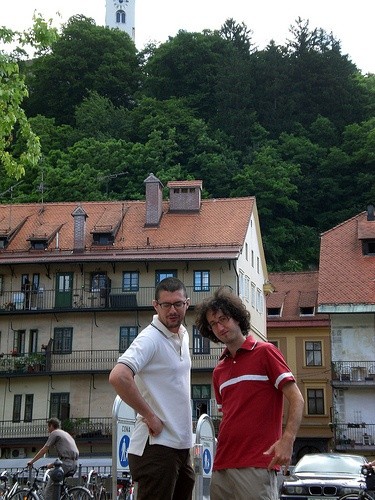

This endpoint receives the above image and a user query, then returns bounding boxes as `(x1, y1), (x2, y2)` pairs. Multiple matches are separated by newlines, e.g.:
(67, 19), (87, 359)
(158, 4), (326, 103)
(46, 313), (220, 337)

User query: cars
(277, 452), (369, 500)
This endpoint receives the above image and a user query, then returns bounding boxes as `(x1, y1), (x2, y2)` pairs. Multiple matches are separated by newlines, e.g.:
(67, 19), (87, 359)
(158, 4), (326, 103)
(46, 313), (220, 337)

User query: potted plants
(61, 416), (89, 439)
(10, 349), (45, 374)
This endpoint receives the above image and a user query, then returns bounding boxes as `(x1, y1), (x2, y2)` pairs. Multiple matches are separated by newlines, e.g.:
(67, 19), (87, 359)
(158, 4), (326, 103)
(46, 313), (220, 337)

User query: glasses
(156, 300), (186, 310)
(208, 316), (231, 330)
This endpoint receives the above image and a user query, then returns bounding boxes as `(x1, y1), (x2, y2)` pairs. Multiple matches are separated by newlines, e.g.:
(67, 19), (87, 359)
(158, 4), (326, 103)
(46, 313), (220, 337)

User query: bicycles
(337, 464), (375, 500)
(0, 463), (135, 500)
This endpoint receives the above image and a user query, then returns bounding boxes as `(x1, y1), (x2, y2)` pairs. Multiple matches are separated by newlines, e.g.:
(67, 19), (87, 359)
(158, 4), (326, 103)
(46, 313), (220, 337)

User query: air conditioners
(350, 367), (367, 383)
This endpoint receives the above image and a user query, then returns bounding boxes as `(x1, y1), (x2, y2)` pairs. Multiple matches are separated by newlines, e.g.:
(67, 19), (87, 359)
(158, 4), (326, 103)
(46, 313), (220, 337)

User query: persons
(109, 276), (194, 500)
(196, 290), (305, 500)
(361, 462), (375, 491)
(27, 417), (79, 500)
(21, 284), (29, 299)
(104, 276), (112, 293)
